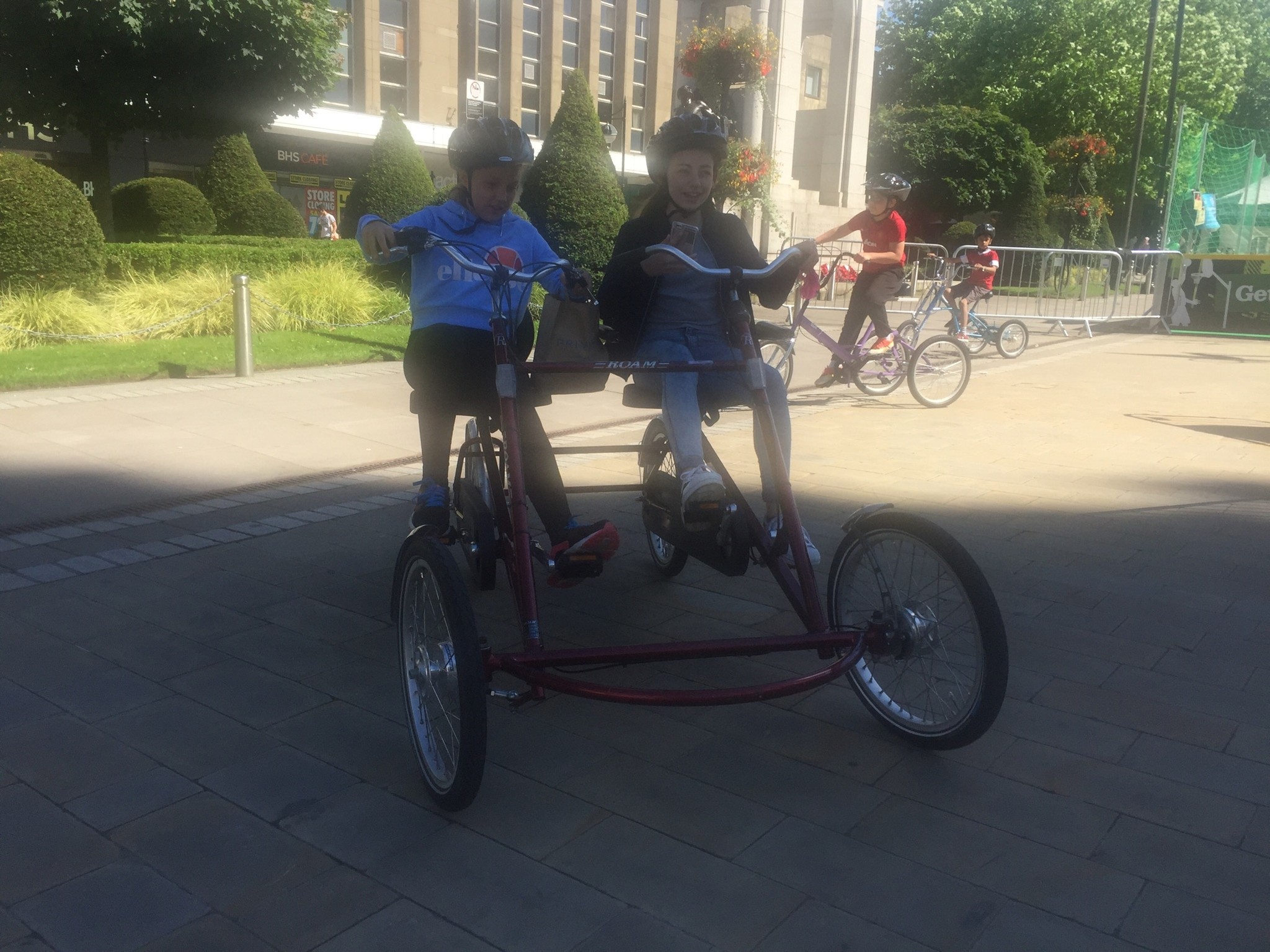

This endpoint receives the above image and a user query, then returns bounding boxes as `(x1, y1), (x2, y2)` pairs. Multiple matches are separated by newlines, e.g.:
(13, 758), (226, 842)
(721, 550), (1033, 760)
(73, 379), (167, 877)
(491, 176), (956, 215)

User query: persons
(315, 203), (338, 240)
(617, 113), (821, 564)
(670, 85), (719, 121)
(358, 115), (620, 588)
(808, 173), (912, 386)
(927, 223), (1000, 341)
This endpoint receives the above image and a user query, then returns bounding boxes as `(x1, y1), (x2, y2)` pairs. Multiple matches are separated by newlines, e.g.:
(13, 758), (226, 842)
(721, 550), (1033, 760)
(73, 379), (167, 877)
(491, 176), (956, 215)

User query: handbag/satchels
(534, 272), (610, 393)
(332, 232), (339, 240)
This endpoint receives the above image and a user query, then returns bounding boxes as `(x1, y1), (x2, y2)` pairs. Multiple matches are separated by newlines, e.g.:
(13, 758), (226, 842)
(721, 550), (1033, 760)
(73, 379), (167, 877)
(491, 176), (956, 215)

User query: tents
(1199, 172), (1270, 255)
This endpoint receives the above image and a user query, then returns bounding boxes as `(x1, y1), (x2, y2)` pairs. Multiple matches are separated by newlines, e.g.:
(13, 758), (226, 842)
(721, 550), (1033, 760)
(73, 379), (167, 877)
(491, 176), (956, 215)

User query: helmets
(975, 224), (996, 239)
(449, 116), (535, 169)
(645, 113), (730, 185)
(861, 172), (912, 201)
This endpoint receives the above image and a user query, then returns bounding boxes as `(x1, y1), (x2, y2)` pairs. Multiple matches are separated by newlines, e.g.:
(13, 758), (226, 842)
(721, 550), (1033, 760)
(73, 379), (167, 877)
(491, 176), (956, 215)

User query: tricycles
(890, 254), (1029, 362)
(754, 251), (973, 410)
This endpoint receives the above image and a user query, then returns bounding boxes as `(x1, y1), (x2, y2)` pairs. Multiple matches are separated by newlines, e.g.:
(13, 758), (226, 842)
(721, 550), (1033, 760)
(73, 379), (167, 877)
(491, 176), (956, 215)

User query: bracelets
(982, 266), (986, 272)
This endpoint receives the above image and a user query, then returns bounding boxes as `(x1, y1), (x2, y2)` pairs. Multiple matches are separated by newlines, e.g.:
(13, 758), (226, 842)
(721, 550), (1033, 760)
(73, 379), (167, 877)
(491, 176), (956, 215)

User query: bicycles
(388, 226), (1013, 814)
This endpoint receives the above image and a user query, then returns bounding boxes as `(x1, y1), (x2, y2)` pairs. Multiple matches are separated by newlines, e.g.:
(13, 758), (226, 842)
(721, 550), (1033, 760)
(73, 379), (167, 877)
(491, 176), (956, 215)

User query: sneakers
(866, 332), (895, 355)
(764, 512), (821, 566)
(680, 463), (726, 532)
(409, 477), (451, 538)
(815, 366), (838, 385)
(954, 333), (969, 341)
(546, 517), (620, 588)
(945, 314), (961, 327)
